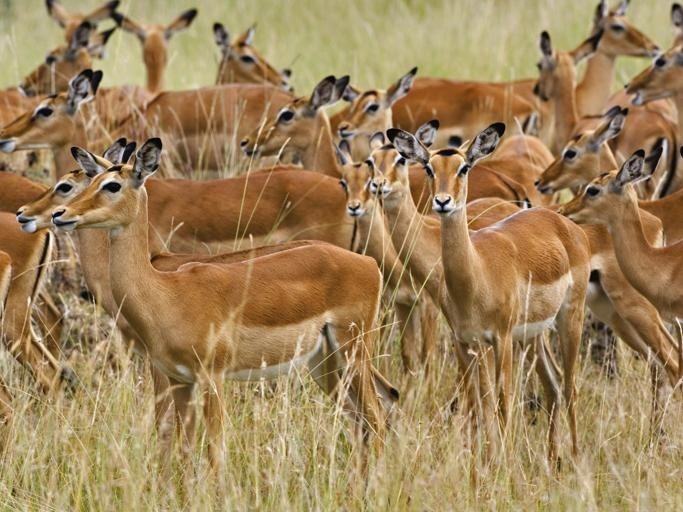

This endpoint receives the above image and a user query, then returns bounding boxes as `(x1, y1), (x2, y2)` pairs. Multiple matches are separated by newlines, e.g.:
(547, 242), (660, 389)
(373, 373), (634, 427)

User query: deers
(1, 0), (683, 478)
